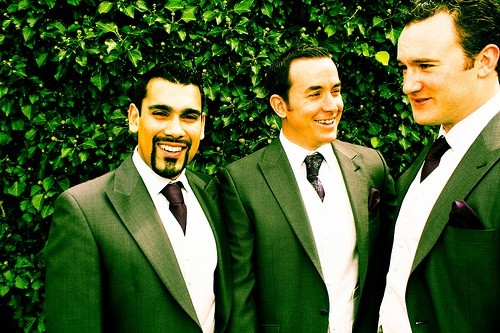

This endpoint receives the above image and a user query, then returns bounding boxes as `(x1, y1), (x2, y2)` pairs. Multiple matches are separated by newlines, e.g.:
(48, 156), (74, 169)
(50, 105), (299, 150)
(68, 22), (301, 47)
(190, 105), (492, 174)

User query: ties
(160, 183), (186, 236)
(304, 154), (325, 203)
(420, 134), (452, 181)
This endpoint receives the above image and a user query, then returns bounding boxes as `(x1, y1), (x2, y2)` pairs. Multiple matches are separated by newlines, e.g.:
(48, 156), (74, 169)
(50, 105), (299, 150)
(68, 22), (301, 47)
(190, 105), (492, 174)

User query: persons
(45, 61), (232, 333)
(353, 0), (500, 333)
(215, 45), (397, 333)
